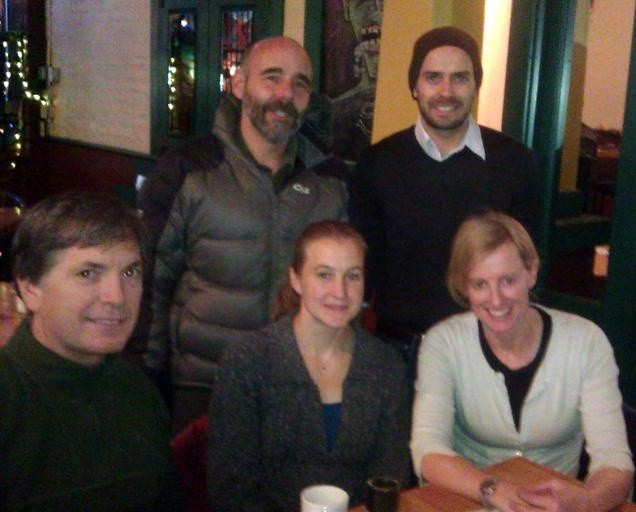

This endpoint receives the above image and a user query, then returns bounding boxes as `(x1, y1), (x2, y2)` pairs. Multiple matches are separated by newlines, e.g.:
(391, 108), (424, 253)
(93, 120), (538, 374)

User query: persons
(127, 33), (358, 428)
(405, 207), (636, 512)
(1, 190), (189, 512)
(205, 218), (413, 512)
(349, 23), (542, 366)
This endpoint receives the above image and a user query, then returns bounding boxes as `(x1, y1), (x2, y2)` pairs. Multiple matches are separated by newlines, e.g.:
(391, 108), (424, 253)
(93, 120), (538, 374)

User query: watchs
(479, 477), (504, 511)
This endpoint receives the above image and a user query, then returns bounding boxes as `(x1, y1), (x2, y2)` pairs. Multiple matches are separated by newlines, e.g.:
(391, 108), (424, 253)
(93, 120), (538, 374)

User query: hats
(409, 27), (482, 92)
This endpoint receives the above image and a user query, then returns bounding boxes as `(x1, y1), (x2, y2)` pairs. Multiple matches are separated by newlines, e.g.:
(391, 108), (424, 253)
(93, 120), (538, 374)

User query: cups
(298, 477), (400, 512)
(592, 244), (609, 277)
